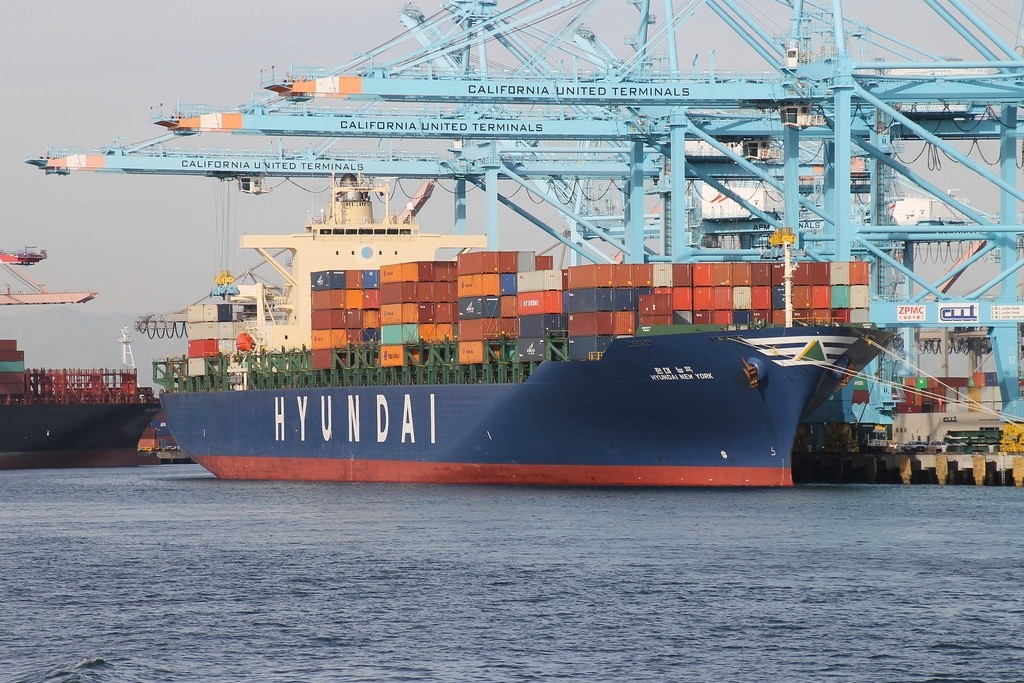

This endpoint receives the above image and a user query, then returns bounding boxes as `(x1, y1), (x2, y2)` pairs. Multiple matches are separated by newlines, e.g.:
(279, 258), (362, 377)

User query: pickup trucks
(901, 439), (946, 453)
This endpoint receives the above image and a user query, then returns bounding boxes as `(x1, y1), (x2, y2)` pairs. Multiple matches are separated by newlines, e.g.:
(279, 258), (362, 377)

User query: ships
(151, 322), (897, 490)
